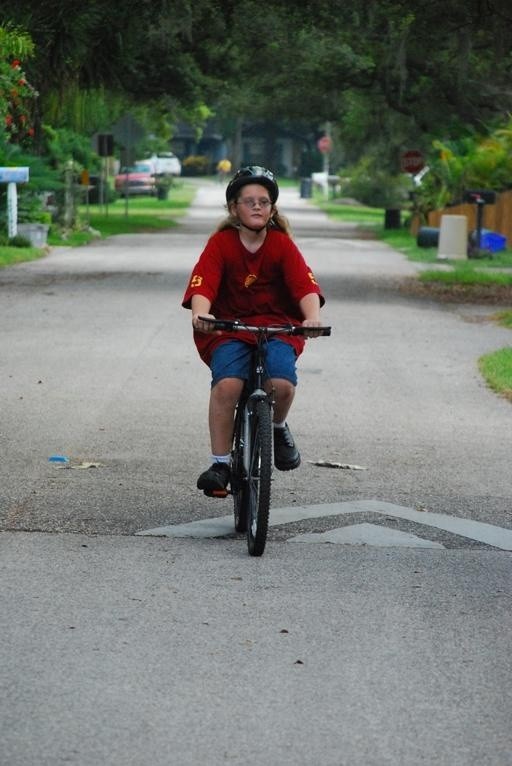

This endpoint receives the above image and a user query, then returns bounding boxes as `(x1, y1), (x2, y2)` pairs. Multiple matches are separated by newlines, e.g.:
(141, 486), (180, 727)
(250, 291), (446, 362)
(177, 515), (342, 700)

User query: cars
(113, 163), (155, 198)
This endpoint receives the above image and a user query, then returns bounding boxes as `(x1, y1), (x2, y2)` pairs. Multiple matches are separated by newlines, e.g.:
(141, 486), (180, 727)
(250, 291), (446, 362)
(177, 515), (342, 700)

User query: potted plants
(17, 196), (51, 248)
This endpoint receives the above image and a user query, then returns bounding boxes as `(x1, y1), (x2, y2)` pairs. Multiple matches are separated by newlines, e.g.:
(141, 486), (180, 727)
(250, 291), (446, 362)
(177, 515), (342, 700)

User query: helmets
(226, 165), (278, 205)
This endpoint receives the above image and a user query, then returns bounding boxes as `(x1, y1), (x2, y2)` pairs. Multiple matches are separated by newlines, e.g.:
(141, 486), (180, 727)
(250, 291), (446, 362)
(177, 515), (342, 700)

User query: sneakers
(274, 421), (301, 471)
(196, 462), (231, 490)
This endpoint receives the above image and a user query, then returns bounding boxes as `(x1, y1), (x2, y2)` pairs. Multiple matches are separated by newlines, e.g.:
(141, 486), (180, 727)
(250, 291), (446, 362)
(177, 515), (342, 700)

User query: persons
(216, 159), (233, 182)
(181, 164), (325, 488)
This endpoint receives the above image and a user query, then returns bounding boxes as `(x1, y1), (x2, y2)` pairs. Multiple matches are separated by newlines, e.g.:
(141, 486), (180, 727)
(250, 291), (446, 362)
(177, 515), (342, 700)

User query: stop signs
(400, 148), (424, 175)
(318, 137), (334, 153)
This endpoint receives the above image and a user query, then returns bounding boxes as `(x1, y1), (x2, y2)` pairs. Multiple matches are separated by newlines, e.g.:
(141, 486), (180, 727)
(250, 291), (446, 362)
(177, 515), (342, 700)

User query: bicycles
(191, 308), (334, 562)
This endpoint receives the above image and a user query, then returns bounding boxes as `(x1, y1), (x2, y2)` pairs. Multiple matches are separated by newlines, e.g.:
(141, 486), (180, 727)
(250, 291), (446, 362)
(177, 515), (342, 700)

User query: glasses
(236, 198), (273, 209)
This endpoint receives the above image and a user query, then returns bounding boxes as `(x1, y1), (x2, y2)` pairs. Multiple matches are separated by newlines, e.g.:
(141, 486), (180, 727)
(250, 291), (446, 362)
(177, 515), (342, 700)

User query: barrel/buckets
(469, 229), (508, 254)
(415, 225), (440, 248)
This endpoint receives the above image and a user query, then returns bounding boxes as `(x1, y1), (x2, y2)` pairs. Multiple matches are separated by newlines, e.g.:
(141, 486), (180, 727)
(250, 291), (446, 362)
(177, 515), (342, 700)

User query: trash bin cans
(158, 184), (168, 199)
(300, 177), (313, 198)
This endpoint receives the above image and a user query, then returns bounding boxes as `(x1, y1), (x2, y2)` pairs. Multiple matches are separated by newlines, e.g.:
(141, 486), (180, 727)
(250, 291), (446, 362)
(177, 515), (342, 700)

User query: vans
(132, 149), (183, 176)
